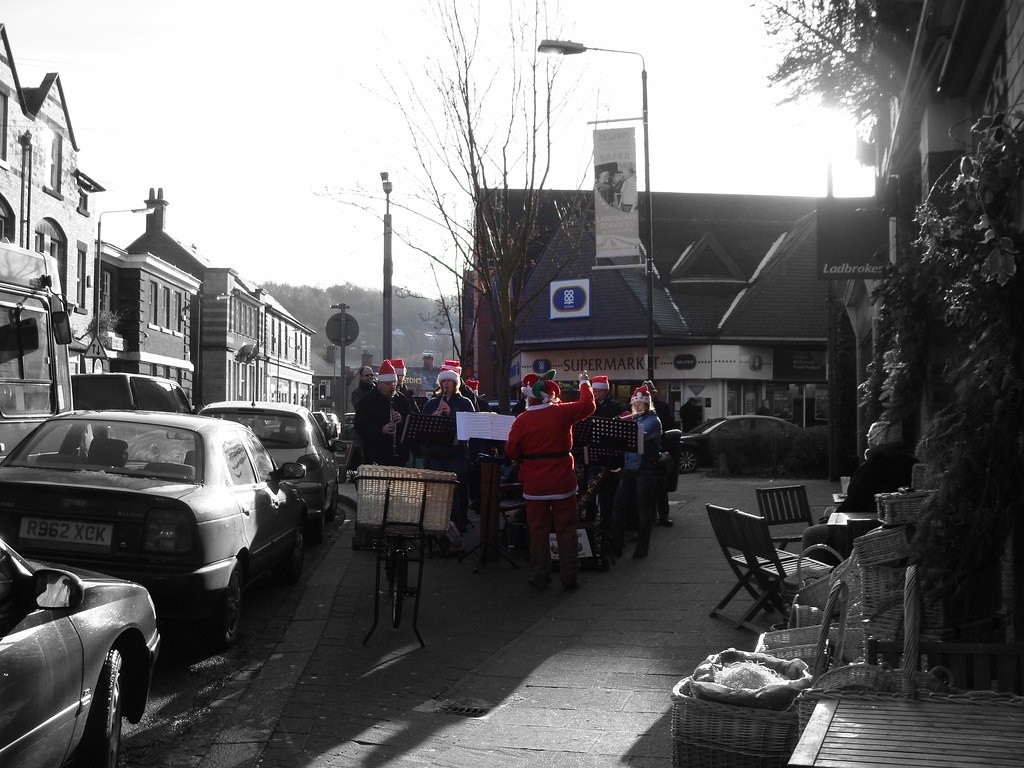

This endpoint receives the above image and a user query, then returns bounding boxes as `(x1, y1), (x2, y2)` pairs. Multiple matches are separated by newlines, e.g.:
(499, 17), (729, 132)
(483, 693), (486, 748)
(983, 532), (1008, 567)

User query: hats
(379, 359), (397, 381)
(630, 385), (651, 403)
(437, 361), (460, 387)
(465, 379), (479, 394)
(526, 380), (561, 410)
(592, 376), (609, 390)
(390, 359), (407, 375)
(643, 381), (657, 392)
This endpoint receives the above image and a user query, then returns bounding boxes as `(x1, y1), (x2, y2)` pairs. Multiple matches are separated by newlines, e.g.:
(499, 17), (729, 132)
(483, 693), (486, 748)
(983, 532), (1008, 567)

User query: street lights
(277, 329), (302, 402)
(537, 39), (655, 393)
(197, 292), (229, 402)
(381, 172), (393, 363)
(96, 207), (156, 338)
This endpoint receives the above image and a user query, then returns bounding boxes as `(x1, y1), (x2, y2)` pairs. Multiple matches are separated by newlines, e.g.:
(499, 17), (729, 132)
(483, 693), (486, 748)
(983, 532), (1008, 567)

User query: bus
(0, 235), (73, 469)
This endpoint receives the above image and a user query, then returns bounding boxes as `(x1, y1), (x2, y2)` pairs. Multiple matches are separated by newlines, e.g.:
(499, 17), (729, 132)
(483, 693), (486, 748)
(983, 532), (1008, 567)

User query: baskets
(761, 410), (969, 660)
(671, 648), (923, 768)
(357, 465), (457, 533)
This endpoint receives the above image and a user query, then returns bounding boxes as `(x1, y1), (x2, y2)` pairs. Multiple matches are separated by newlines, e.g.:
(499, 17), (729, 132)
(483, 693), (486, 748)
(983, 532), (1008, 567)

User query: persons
(503, 369), (597, 592)
(419, 366), (477, 537)
(831, 418), (922, 535)
(609, 384), (663, 559)
(351, 358), (673, 560)
(354, 360), (408, 465)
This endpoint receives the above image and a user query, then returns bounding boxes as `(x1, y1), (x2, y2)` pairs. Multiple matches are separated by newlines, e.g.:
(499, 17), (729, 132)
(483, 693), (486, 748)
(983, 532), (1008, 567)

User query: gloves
(579, 370), (590, 381)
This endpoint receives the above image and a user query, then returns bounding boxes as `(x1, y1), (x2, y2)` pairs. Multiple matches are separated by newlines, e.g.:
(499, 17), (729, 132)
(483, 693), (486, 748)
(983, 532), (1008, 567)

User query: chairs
(184, 451), (196, 467)
(869, 638), (1024, 698)
(706, 483), (835, 634)
(145, 461), (195, 478)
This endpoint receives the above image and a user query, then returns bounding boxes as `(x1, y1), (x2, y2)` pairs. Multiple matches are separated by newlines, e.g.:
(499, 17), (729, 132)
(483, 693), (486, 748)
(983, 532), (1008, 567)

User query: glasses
(362, 374), (373, 377)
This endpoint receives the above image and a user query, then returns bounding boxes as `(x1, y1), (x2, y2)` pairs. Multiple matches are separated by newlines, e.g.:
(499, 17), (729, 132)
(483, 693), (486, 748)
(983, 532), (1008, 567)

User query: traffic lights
(319, 384), (326, 400)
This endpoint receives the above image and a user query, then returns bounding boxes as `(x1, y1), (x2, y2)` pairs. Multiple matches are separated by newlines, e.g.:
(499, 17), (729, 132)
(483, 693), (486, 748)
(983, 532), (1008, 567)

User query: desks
(827, 512), (879, 526)
(787, 699), (1024, 768)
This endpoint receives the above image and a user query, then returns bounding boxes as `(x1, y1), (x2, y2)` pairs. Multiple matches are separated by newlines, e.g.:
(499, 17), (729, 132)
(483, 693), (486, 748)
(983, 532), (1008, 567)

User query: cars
(0, 534), (162, 768)
(326, 413), (341, 439)
(675, 414), (803, 474)
(198, 401), (346, 545)
(345, 412), (356, 438)
(0, 409), (306, 649)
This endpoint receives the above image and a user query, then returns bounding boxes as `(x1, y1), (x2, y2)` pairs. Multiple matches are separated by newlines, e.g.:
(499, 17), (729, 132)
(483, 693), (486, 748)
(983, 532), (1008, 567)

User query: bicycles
(350, 469), (428, 630)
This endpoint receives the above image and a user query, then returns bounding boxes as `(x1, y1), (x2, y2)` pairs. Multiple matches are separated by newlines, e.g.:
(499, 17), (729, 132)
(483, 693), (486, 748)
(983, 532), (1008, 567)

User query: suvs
(309, 409), (332, 442)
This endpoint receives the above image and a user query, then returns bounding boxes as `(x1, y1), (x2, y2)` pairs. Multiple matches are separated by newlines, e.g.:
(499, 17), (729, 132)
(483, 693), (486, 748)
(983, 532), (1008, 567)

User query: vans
(71, 373), (195, 415)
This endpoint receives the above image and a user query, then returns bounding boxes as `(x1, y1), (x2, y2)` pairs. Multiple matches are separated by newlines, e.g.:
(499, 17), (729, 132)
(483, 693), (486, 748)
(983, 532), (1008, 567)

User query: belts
(522, 451), (570, 460)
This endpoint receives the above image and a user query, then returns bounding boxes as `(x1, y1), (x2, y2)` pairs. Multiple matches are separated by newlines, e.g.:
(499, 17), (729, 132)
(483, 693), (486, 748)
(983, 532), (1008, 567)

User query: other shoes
(660, 519), (673, 526)
(538, 573), (552, 590)
(563, 581), (579, 590)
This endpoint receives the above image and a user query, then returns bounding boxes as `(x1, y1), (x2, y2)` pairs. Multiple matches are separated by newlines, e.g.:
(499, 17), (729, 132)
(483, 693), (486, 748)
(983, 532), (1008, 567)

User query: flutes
(436, 387), (448, 417)
(620, 410), (645, 419)
(389, 389), (399, 458)
